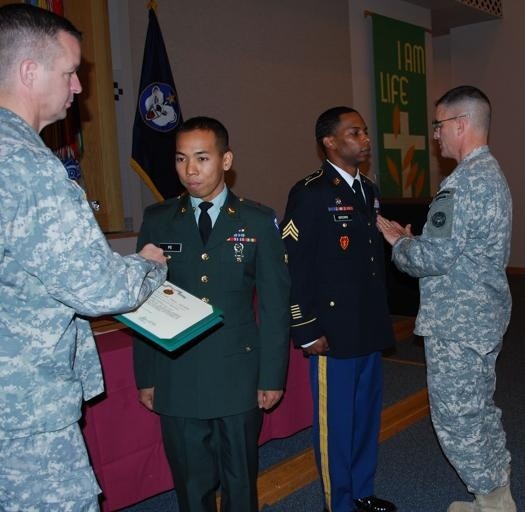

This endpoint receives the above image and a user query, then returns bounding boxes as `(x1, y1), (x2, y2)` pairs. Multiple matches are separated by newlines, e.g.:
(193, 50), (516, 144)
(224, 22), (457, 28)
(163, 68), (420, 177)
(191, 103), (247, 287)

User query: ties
(353, 180), (365, 205)
(197, 202), (213, 245)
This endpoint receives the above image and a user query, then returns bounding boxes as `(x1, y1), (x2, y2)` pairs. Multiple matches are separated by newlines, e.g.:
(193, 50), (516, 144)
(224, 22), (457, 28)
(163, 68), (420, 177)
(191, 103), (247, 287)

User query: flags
(128, 2), (186, 209)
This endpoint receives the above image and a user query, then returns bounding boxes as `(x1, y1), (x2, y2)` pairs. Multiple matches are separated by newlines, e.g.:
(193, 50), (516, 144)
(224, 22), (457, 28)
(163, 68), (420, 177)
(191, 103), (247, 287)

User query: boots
(447, 466), (517, 512)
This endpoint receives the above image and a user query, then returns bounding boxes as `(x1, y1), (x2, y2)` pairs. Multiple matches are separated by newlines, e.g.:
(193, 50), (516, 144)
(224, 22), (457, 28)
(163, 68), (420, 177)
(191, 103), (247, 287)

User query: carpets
(78, 339), (311, 512)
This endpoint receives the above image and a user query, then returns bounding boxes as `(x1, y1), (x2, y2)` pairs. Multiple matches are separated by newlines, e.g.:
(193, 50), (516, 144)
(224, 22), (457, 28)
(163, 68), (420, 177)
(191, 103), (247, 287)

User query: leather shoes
(355, 495), (397, 511)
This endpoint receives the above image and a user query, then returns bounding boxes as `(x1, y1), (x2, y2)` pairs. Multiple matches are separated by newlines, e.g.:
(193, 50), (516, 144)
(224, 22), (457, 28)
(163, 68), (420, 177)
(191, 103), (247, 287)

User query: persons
(0, 1), (169, 511)
(132, 116), (295, 512)
(375, 82), (520, 512)
(279, 105), (402, 511)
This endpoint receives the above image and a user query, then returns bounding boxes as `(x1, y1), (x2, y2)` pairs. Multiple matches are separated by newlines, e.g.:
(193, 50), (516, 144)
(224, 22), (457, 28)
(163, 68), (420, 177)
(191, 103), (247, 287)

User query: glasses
(431, 115), (463, 132)
